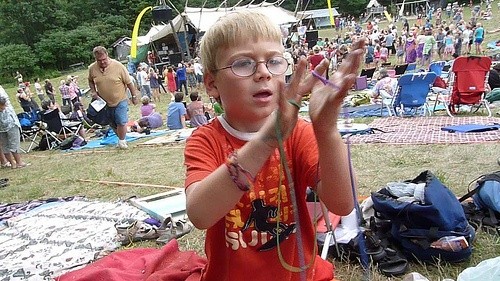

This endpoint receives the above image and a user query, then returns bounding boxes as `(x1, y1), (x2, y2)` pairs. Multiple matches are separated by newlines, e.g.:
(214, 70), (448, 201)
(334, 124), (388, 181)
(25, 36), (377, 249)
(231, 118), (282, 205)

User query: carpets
(298, 86), (447, 117)
(342, 116), (500, 145)
(55, 237), (207, 281)
(57, 129), (169, 153)
(135, 127), (198, 148)
(0, 196), (162, 281)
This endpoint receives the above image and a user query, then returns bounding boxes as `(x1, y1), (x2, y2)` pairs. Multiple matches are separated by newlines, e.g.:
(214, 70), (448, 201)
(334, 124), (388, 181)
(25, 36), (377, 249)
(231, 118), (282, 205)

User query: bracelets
(133, 94), (137, 98)
(226, 149), (256, 192)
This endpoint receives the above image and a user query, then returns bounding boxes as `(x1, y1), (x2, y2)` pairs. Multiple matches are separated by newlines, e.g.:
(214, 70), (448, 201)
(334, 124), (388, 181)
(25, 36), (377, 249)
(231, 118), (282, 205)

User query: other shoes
(116, 140), (128, 149)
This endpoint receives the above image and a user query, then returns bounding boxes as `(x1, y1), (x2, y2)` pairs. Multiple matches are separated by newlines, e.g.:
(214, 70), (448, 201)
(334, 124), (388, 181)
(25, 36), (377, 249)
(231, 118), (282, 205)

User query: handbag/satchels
(370, 169), (476, 265)
(87, 92), (114, 125)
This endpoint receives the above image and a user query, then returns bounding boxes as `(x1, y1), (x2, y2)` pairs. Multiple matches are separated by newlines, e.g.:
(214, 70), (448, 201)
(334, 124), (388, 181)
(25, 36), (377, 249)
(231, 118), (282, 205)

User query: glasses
(210, 55), (291, 77)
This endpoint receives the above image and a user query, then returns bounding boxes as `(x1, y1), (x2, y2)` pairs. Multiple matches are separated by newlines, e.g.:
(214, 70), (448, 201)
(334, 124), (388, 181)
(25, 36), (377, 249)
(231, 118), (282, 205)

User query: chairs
(14, 108), (42, 153)
(350, 60), (451, 90)
(433, 55), (493, 117)
(39, 107), (84, 151)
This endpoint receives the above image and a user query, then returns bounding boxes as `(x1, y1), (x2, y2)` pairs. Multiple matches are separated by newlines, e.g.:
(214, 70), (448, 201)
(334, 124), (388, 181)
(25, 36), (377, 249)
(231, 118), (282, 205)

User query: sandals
(333, 229), (408, 276)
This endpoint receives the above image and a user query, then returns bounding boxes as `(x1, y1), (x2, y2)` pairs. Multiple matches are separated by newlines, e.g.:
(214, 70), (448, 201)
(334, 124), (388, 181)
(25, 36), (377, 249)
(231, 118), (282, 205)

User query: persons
(88, 45), (138, 150)
(284, 0), (499, 104)
(13, 70), (94, 133)
(0, 85), (31, 169)
(185, 11), (366, 281)
(125, 42), (208, 133)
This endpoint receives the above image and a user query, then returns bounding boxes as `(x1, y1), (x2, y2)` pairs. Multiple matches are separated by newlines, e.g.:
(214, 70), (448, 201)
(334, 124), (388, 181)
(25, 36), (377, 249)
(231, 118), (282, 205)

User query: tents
(125, 2), (302, 66)
